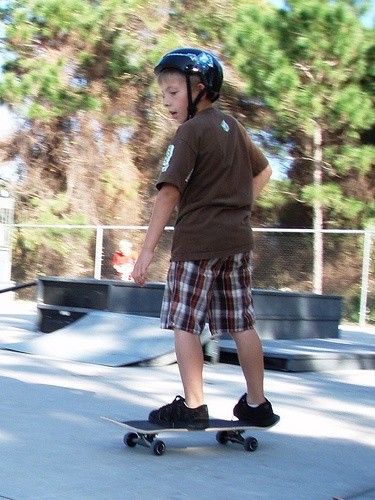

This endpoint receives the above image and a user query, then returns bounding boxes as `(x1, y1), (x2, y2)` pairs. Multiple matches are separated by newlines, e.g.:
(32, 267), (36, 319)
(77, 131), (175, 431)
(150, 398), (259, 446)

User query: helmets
(154, 48), (223, 103)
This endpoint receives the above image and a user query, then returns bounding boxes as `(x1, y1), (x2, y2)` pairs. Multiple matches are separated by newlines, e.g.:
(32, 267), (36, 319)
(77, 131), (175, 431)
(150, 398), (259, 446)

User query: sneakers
(148, 395), (209, 429)
(233, 392), (273, 426)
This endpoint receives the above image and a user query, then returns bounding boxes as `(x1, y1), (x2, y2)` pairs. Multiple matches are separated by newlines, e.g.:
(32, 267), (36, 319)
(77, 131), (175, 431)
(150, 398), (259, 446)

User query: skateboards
(100, 415), (280, 455)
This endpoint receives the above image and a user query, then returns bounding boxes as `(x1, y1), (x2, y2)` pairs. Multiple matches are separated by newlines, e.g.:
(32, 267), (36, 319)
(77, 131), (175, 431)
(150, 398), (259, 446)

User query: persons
(131, 47), (275, 430)
